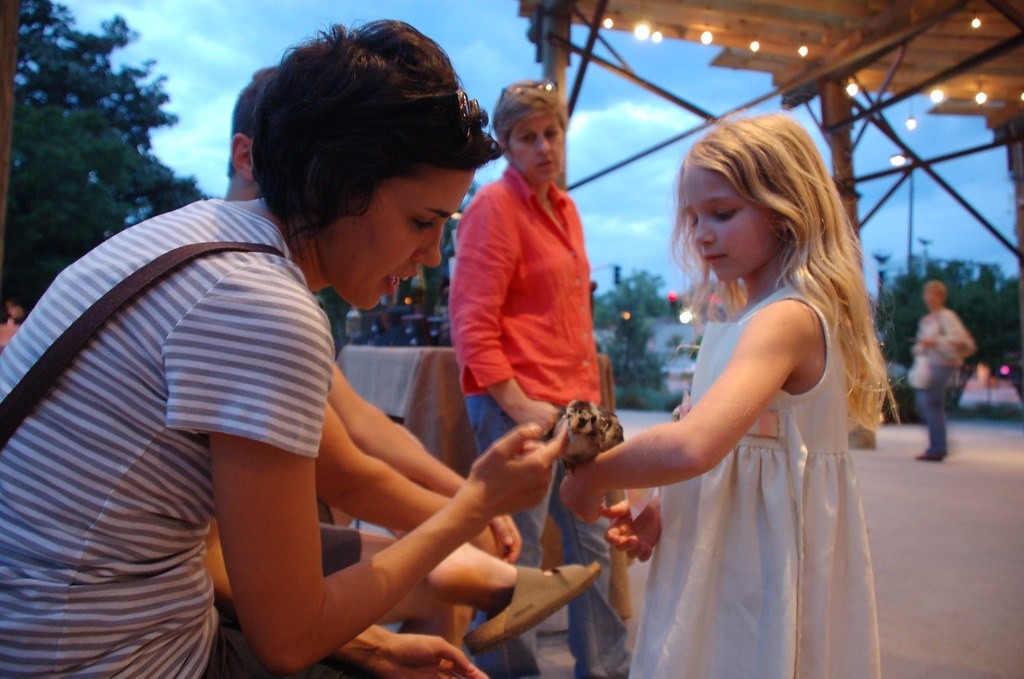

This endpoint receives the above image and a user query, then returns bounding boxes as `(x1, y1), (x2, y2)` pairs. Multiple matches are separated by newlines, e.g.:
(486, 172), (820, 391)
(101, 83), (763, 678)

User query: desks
(339, 345), (632, 624)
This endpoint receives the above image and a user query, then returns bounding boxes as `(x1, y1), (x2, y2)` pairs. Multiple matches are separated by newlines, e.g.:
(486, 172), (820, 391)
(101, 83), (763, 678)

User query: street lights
(892, 152), (914, 273)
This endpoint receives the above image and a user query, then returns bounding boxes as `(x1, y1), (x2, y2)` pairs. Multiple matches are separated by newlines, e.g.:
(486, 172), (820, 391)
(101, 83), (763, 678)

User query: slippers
(462, 561), (601, 656)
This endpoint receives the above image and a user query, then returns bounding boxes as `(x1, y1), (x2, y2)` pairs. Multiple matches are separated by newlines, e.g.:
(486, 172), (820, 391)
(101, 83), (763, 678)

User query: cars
(661, 352), (695, 390)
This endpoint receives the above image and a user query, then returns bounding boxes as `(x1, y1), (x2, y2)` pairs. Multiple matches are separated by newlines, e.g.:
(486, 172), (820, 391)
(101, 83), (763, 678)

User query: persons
(448, 82), (630, 679)
(910, 279), (977, 461)
(0, 19), (604, 679)
(560, 113), (899, 679)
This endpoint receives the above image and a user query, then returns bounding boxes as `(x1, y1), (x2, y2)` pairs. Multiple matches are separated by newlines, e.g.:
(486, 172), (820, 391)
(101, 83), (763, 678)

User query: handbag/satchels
(907, 353), (932, 389)
(939, 309), (976, 359)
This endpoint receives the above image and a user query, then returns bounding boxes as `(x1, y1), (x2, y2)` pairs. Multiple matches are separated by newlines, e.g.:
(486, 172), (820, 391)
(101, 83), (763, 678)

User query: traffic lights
(667, 292), (676, 314)
(614, 266), (621, 284)
(621, 310), (631, 337)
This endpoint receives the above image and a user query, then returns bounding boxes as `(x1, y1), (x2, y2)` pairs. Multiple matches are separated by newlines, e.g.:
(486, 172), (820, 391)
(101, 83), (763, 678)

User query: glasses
(500, 80), (557, 104)
(451, 90), (480, 139)
(7, 315), (25, 326)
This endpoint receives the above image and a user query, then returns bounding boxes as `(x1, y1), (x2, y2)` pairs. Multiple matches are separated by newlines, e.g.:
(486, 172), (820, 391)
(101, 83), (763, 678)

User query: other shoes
(916, 453), (944, 461)
